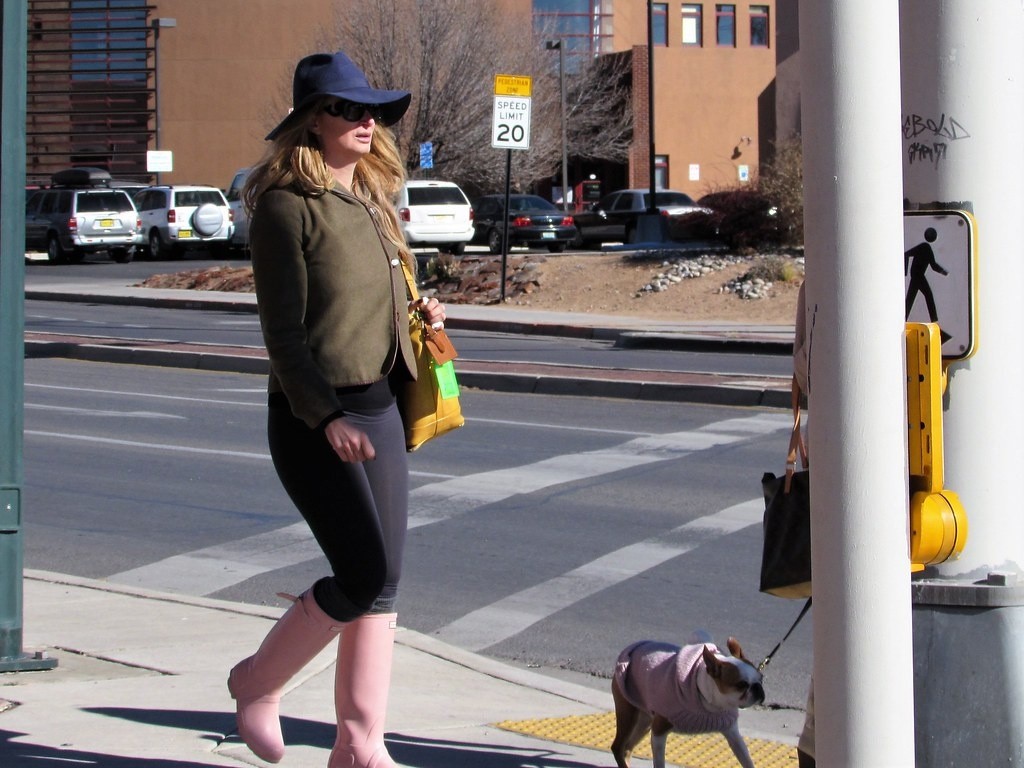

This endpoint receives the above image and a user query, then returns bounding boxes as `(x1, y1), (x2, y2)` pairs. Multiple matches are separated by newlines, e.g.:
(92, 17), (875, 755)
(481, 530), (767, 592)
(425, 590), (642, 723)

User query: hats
(264, 52), (412, 141)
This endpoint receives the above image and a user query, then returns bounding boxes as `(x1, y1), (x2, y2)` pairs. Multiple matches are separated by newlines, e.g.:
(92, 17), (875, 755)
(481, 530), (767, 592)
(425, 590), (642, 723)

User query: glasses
(312, 101), (381, 125)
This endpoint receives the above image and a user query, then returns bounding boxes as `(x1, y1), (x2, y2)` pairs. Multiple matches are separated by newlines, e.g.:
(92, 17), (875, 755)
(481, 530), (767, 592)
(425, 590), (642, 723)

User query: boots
(327, 609), (401, 768)
(226, 581), (354, 764)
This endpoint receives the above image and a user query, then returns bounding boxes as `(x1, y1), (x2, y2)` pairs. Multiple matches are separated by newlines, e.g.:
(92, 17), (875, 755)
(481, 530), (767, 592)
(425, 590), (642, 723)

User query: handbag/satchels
(386, 308), (465, 453)
(761, 372), (813, 599)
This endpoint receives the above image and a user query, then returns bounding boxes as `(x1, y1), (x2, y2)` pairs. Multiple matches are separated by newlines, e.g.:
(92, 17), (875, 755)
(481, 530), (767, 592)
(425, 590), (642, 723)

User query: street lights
(152, 16), (178, 187)
(545, 37), (569, 217)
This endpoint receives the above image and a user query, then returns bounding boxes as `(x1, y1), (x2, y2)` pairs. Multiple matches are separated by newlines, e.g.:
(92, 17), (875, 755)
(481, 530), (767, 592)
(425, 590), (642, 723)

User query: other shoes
(796, 747), (816, 768)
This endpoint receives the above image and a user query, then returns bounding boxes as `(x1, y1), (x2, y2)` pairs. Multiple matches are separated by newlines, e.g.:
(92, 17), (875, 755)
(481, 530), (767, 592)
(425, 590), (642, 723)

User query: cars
(387, 177), (475, 255)
(694, 189), (785, 248)
(575, 189), (718, 252)
(470, 193), (578, 259)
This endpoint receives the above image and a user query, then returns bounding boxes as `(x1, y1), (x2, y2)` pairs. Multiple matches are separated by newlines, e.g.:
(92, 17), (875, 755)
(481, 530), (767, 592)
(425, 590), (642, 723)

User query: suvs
(25, 167), (145, 264)
(99, 180), (159, 213)
(220, 166), (262, 260)
(131, 184), (236, 261)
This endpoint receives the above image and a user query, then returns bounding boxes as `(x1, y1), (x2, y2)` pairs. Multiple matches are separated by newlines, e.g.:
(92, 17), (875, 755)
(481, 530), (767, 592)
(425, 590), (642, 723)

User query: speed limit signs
(493, 121), (529, 147)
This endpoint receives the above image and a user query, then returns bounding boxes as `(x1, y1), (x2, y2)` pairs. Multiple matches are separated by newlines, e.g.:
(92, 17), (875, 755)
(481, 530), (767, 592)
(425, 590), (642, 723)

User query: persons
(228, 52), (446, 768)
(794, 279), (816, 768)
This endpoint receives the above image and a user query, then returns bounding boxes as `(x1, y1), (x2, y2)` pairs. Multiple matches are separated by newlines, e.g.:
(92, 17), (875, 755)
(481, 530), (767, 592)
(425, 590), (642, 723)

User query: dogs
(611, 635), (765, 768)
